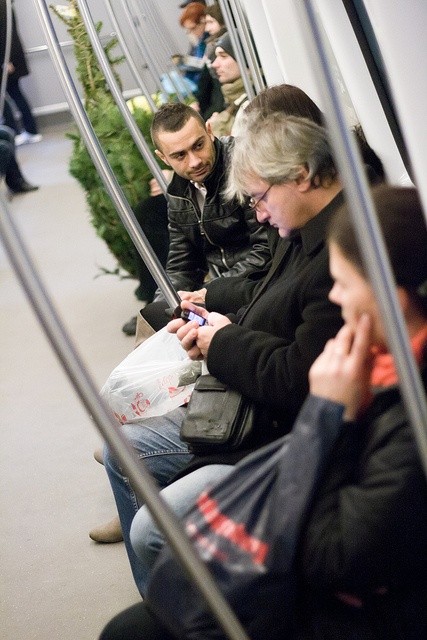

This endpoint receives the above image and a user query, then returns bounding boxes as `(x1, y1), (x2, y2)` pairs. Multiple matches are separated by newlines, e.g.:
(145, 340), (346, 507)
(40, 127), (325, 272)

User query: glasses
(248, 184), (273, 212)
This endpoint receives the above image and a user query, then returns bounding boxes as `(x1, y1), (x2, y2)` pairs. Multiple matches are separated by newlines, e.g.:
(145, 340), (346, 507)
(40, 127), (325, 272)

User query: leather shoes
(122, 316), (136, 333)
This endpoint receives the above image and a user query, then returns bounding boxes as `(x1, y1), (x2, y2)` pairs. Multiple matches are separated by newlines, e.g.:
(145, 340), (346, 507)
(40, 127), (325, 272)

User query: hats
(216, 28), (262, 69)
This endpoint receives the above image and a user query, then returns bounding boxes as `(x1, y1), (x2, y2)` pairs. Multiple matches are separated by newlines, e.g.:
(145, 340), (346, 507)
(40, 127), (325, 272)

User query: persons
(179, 0), (229, 116)
(102, 112), (381, 599)
(89, 84), (384, 544)
(0, 126), (39, 193)
(0, 0), (43, 146)
(134, 102), (280, 350)
(123, 28), (265, 336)
(99, 184), (427, 640)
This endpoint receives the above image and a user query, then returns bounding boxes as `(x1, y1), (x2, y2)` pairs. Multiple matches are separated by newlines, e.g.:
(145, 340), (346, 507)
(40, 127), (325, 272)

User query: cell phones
(180, 308), (209, 330)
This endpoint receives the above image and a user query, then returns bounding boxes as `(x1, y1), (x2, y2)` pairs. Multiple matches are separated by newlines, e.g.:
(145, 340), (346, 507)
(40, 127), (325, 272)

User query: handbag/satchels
(138, 435), (290, 639)
(180, 374), (253, 453)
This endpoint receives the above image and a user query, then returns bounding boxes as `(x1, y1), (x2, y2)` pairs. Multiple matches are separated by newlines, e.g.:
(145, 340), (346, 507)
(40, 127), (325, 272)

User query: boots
(90, 511), (124, 543)
(94, 311), (157, 465)
